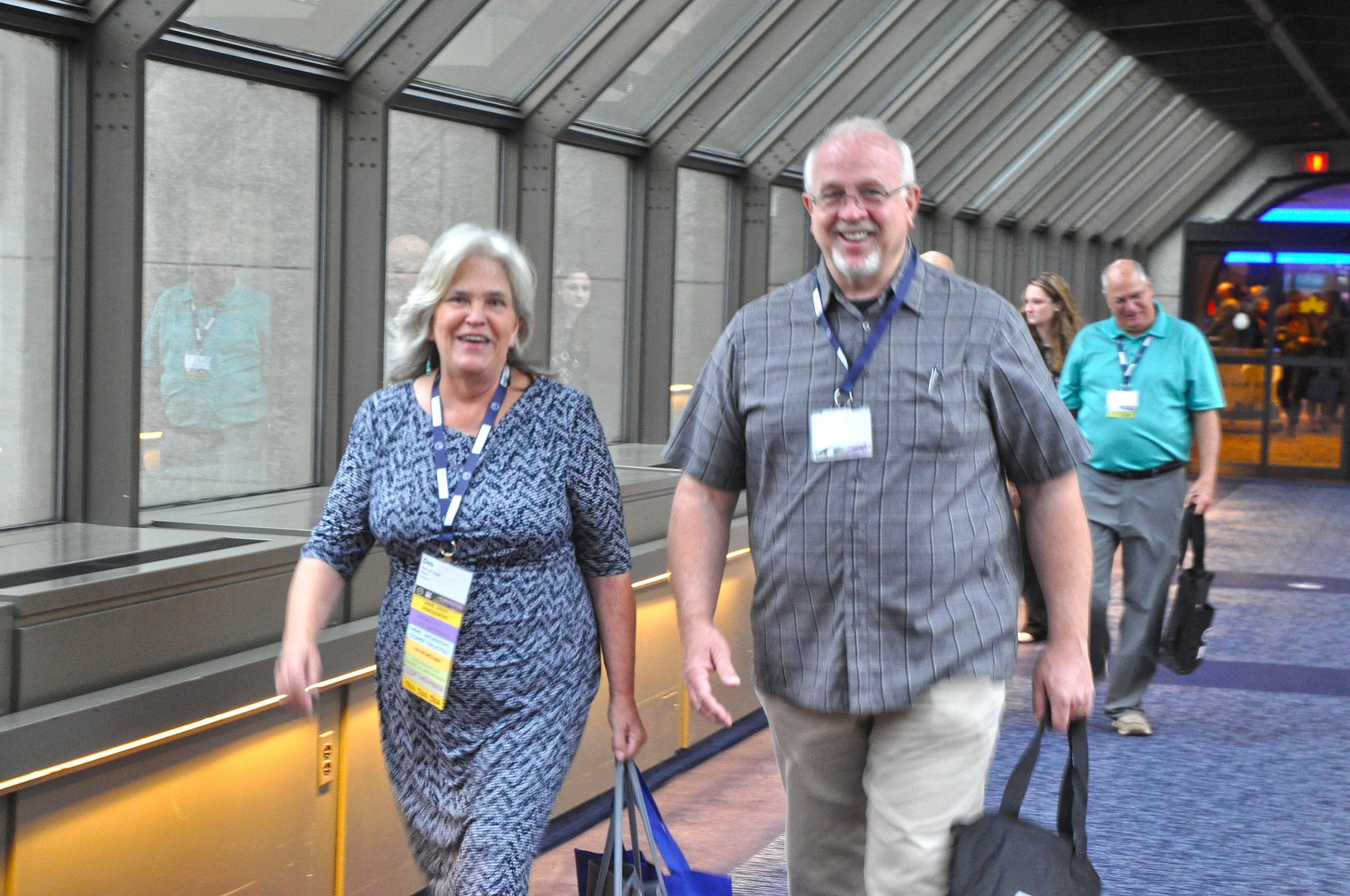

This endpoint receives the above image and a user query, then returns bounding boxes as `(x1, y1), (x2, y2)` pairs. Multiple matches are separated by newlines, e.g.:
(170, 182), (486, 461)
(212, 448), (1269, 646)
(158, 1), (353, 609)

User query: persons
(143, 232), (275, 499)
(381, 233), (424, 378)
(1202, 278), (1350, 436)
(919, 250), (956, 275)
(1017, 272), (1091, 641)
(273, 222), (647, 896)
(659, 114), (1098, 896)
(551, 262), (613, 398)
(1053, 256), (1226, 733)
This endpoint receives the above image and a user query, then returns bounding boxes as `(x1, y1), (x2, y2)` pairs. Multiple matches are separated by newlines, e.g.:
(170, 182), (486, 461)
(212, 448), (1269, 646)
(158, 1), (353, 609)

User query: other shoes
(1018, 626), (1045, 642)
(1111, 714), (1151, 735)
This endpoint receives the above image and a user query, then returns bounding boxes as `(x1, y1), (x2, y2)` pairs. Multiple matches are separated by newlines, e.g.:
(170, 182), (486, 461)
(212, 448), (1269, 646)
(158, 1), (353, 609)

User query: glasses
(813, 181), (908, 207)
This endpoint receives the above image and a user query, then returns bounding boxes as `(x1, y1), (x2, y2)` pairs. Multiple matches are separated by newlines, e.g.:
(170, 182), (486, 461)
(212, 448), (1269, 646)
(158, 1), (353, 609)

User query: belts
(1106, 461), (1186, 479)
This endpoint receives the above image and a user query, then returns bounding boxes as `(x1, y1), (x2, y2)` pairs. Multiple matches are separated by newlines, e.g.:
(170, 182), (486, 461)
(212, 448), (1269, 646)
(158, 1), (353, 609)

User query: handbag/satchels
(587, 758), (667, 896)
(1160, 507), (1214, 674)
(949, 713), (1101, 896)
(574, 758), (732, 896)
